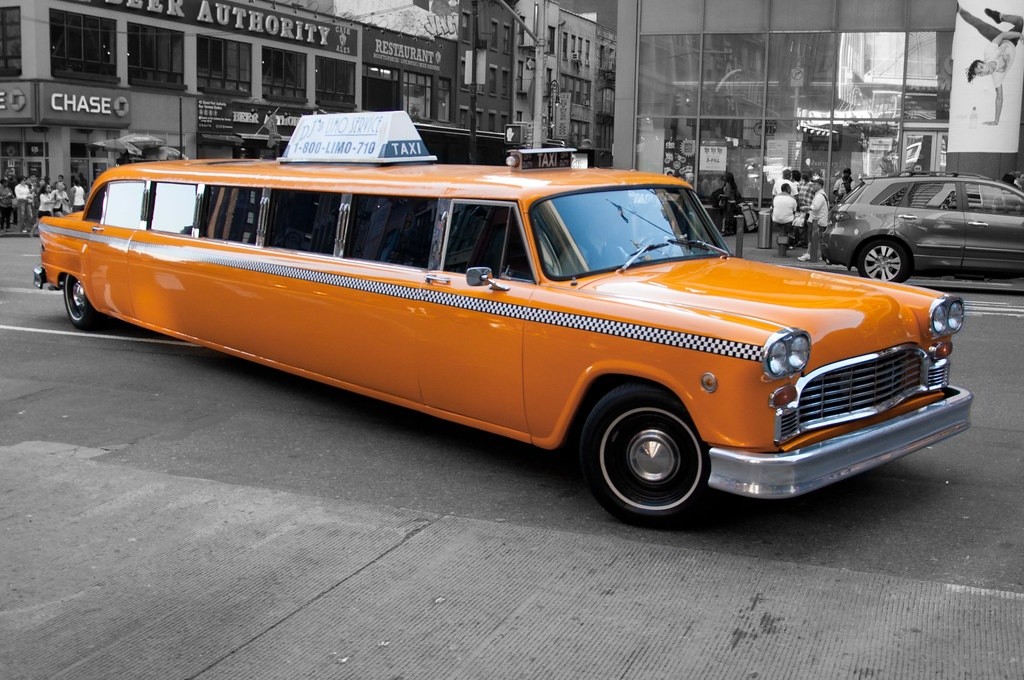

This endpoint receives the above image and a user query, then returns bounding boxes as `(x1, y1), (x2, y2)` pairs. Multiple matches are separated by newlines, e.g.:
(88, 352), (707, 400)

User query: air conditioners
(572, 53), (581, 61)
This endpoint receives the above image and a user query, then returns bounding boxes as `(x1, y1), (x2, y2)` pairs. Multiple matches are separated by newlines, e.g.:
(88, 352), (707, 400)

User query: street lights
(545, 78), (562, 142)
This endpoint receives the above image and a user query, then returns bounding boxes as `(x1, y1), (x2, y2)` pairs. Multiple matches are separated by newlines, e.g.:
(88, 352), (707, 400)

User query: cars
(32, 109), (975, 533)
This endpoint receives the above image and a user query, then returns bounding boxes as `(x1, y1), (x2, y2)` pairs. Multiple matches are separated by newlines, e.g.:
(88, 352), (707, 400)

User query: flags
(264, 113), (278, 148)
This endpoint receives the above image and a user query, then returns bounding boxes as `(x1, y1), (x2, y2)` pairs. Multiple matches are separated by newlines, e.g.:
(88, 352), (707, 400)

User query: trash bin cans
(757, 211), (772, 248)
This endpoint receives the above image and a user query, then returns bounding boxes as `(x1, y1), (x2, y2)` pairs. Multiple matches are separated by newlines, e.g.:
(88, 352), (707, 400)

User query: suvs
(818, 168), (1024, 283)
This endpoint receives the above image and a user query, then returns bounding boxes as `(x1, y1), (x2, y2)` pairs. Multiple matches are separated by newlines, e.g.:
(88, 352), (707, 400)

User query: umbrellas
(144, 147), (187, 160)
(119, 132), (164, 149)
(86, 137), (142, 165)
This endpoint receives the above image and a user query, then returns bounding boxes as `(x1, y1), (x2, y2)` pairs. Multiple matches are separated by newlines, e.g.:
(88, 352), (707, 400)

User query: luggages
(741, 206), (758, 233)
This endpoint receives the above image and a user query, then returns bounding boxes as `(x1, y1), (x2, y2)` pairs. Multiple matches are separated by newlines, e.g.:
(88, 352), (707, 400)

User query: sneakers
(798, 253), (810, 262)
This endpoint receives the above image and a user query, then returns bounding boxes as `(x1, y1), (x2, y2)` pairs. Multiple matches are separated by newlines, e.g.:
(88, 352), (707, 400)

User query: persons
(1002, 167), (1024, 192)
(71, 176), (76, 187)
(30, 184), (54, 238)
(956, 2), (1024, 126)
(51, 175), (67, 189)
(797, 169), (814, 248)
(797, 179), (830, 261)
(14, 176), (34, 232)
(702, 175), (722, 195)
(0, 174), (52, 232)
(666, 171), (672, 175)
(772, 169), (801, 210)
(69, 179), (85, 212)
(832, 168), (857, 202)
(50, 182), (69, 217)
(773, 183), (797, 250)
(78, 173), (88, 192)
(718, 173), (737, 236)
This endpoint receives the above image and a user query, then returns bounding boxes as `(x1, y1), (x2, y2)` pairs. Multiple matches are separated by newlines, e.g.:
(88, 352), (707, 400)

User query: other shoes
(6, 229), (11, 231)
(796, 242), (806, 248)
(20, 229), (27, 233)
(722, 233), (733, 236)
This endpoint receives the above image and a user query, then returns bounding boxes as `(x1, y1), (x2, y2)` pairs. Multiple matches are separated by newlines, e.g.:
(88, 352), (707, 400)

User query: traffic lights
(503, 122), (525, 146)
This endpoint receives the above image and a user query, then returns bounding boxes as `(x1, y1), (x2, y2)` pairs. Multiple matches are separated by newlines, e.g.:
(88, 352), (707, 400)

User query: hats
(811, 178), (823, 186)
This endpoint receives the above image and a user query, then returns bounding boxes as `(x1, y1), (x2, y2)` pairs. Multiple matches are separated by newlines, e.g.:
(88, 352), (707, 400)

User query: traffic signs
(525, 55), (536, 72)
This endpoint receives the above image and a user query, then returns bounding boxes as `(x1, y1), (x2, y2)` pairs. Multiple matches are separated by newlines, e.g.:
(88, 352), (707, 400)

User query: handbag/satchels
(12, 198), (18, 208)
(791, 213), (806, 227)
(62, 204), (71, 215)
